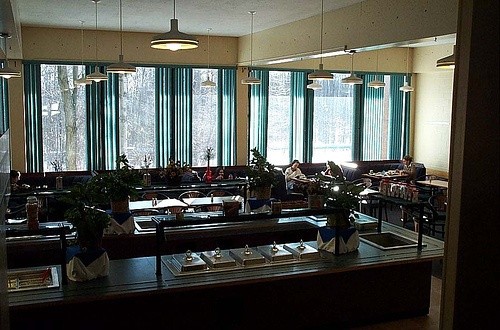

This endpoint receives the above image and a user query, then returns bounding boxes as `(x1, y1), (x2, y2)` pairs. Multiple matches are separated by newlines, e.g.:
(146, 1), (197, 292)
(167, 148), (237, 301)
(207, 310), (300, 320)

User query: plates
(369, 173), (384, 176)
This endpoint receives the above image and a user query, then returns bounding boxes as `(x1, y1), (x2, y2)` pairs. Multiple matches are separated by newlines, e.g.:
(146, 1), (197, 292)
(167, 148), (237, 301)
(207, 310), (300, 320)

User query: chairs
(206, 190), (235, 211)
(139, 192), (171, 216)
(178, 190), (206, 213)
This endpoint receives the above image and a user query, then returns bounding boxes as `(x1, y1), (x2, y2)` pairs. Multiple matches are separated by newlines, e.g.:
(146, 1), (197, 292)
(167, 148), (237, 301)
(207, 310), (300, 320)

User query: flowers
(158, 159), (192, 179)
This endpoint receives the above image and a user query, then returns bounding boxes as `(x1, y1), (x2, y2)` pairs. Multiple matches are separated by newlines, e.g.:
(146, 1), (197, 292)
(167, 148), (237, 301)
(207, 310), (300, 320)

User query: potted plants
(306, 179), (326, 209)
(102, 153), (143, 215)
(246, 147), (280, 199)
(315, 161), (371, 227)
(64, 174), (112, 253)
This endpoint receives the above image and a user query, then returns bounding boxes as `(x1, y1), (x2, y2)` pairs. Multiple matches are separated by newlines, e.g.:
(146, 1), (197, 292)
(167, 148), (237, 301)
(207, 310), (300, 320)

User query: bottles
(379, 178), (419, 202)
(25, 196), (39, 225)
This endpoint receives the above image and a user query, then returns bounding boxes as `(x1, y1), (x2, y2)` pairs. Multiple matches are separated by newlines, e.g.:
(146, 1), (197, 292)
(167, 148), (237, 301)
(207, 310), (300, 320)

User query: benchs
(12, 159), (428, 184)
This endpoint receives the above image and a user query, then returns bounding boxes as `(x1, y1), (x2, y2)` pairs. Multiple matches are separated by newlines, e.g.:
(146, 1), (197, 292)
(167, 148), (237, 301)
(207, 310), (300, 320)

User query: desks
(5, 172), (450, 330)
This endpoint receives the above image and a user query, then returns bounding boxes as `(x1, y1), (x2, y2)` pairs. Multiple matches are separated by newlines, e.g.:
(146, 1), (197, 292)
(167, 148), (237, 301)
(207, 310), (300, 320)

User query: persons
(324, 161), (344, 180)
(10, 169), (30, 193)
(396, 156), (416, 181)
(284, 160), (305, 193)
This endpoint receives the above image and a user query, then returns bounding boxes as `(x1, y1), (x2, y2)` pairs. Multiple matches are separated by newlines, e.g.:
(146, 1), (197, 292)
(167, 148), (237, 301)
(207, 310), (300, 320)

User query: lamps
(0, 33), (21, 79)
(399, 47), (414, 92)
(201, 28), (216, 87)
(241, 10), (261, 84)
(150, 0), (199, 51)
(74, 0), (136, 86)
(367, 50), (385, 89)
(307, 68), (322, 90)
(308, 0), (333, 80)
(341, 51), (363, 85)
(436, 44), (455, 69)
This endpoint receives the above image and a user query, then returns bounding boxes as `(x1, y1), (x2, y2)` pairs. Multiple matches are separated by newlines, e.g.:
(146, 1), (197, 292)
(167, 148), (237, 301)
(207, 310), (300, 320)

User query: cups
(370, 170), (373, 174)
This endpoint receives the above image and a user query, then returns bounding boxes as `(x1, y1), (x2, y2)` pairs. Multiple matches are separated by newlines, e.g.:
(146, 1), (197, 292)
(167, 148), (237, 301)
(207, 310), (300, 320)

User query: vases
(166, 176), (182, 189)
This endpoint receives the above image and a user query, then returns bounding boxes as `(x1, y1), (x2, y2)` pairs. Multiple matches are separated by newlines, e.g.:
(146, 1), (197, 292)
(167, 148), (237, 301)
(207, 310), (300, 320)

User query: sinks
(358, 230), (427, 251)
